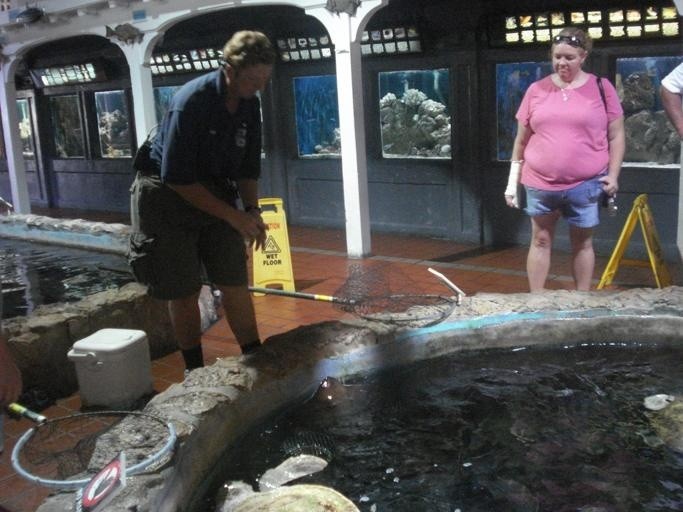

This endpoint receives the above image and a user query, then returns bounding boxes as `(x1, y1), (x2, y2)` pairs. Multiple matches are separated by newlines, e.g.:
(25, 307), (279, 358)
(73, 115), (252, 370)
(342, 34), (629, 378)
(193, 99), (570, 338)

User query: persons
(659, 61), (683, 262)
(128, 30), (276, 373)
(0, 327), (24, 512)
(503, 28), (625, 294)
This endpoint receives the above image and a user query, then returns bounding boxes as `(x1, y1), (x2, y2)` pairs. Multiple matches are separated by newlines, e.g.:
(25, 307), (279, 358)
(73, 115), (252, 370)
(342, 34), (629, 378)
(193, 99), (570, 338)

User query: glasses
(554, 35), (586, 49)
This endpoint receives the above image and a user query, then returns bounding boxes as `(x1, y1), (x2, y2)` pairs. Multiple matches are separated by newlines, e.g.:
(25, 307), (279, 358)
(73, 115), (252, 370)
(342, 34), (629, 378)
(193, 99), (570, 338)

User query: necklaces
(556, 74), (582, 103)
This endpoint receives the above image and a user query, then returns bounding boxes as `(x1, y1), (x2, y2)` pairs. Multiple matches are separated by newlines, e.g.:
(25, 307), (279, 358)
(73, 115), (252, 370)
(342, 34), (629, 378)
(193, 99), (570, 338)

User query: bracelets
(510, 160), (524, 164)
(244, 205), (263, 215)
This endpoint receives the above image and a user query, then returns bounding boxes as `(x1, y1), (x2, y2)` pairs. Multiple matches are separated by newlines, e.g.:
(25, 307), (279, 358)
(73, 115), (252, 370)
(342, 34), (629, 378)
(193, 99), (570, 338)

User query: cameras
(602, 192), (618, 215)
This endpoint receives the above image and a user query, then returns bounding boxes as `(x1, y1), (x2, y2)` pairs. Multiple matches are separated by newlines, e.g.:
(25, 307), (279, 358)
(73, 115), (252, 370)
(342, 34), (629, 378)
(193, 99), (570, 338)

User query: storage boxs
(65, 325), (155, 410)
